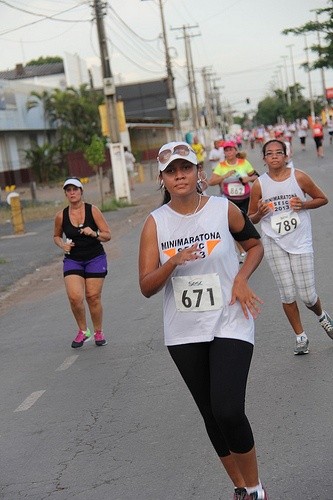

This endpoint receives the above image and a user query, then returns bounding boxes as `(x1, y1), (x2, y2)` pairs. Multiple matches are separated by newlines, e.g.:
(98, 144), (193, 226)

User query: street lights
(266, 43), (298, 106)
(299, 32), (315, 125)
(310, 8), (331, 128)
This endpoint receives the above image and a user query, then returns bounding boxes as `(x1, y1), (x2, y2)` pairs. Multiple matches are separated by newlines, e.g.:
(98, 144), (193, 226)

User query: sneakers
(94, 331), (108, 346)
(293, 336), (310, 355)
(71, 328), (91, 349)
(318, 311), (333, 340)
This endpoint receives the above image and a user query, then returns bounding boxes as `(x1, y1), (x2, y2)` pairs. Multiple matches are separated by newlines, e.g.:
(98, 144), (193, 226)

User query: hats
(223, 141), (236, 148)
(63, 179), (84, 191)
(157, 141), (197, 171)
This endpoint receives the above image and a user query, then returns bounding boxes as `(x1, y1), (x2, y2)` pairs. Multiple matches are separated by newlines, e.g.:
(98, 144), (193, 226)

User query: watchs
(94, 231), (100, 239)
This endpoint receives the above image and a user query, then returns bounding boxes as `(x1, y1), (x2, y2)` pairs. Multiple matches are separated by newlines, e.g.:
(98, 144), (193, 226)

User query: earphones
(161, 179), (163, 183)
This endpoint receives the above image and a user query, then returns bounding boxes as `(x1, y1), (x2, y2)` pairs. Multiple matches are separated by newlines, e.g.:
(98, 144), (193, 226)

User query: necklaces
(193, 194), (202, 213)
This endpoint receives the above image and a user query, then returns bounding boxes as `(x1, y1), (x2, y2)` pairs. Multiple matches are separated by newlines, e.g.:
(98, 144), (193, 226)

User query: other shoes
(231, 486), (267, 500)
(239, 253), (246, 263)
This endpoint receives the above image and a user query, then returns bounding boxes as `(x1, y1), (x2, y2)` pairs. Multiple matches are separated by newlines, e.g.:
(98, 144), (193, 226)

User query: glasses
(158, 145), (197, 164)
(263, 152), (285, 157)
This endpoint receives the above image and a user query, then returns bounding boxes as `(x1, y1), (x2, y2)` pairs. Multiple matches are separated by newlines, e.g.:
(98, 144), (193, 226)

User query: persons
(137, 141), (266, 500)
(247, 139), (333, 351)
(55, 177), (111, 348)
(210, 115), (333, 263)
(192, 137), (207, 176)
